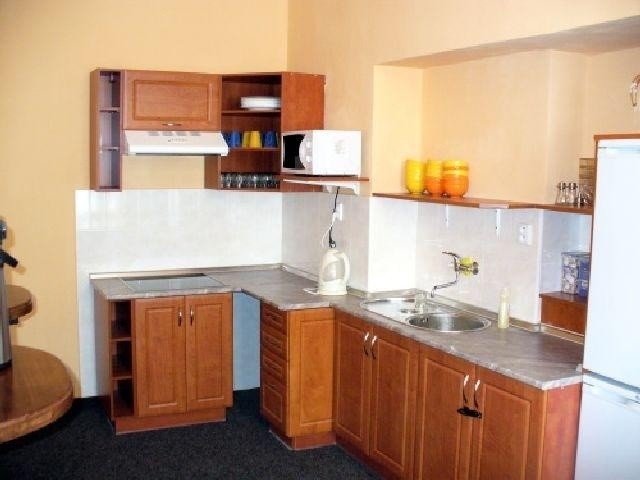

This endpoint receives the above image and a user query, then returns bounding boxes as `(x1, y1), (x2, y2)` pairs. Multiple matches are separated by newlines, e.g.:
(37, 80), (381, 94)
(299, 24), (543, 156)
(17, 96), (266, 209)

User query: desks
(4, 282), (33, 323)
(0, 345), (74, 444)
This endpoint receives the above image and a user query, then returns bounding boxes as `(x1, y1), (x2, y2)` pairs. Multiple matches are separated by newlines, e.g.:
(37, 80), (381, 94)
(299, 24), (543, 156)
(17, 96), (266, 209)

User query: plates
(239, 96), (280, 112)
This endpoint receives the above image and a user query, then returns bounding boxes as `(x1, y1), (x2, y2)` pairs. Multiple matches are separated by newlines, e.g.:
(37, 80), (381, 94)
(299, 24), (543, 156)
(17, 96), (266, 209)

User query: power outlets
(332, 203), (343, 222)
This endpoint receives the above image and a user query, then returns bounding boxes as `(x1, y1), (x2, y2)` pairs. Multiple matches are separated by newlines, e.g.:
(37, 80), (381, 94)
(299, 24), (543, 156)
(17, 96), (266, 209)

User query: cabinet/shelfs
(260, 302), (336, 450)
(203, 73), (326, 192)
(539, 201), (599, 337)
(123, 70), (222, 132)
(418, 343), (581, 480)
(88, 69), (122, 193)
(337, 310), (418, 480)
(95, 288), (232, 437)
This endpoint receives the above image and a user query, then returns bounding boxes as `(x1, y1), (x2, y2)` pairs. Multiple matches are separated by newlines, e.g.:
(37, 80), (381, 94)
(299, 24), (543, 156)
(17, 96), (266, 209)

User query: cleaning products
(497, 287), (511, 330)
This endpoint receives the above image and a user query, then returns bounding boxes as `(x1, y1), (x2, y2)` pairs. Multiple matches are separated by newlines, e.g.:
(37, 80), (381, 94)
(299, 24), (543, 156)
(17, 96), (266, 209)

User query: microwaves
(281, 130), (361, 176)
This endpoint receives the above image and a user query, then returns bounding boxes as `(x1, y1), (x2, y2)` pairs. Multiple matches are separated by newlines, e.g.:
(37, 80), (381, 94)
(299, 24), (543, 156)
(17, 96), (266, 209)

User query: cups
(219, 128), (279, 150)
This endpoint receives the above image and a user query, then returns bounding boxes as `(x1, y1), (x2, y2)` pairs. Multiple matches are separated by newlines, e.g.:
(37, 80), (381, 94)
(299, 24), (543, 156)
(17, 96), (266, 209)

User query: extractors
(123, 129), (228, 156)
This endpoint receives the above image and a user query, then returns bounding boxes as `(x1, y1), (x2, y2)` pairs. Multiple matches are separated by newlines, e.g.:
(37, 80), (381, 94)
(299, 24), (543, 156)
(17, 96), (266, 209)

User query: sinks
(404, 311), (492, 333)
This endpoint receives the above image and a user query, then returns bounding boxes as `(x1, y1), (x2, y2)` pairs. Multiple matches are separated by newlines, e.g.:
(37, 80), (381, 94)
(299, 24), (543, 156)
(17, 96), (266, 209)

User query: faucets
(431, 251), (459, 298)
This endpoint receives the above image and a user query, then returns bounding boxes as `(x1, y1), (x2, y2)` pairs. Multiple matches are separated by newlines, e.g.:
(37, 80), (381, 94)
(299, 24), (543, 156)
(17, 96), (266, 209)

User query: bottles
(219, 171), (281, 189)
(498, 288), (510, 328)
(556, 182), (594, 207)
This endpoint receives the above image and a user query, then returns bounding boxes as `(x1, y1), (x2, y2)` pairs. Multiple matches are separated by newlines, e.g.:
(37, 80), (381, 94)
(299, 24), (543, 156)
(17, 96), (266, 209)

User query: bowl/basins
(405, 160), (469, 199)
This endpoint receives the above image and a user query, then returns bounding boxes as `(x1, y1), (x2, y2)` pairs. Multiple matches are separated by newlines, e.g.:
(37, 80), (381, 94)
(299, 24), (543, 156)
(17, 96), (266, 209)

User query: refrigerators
(573, 138), (640, 480)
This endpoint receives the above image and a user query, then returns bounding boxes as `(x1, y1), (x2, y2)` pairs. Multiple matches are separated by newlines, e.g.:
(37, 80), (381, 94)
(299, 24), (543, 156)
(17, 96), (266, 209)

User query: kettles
(318, 249), (350, 296)
